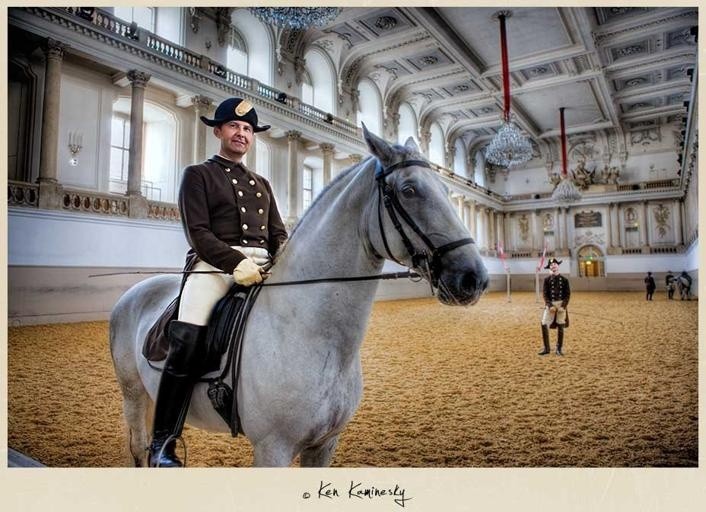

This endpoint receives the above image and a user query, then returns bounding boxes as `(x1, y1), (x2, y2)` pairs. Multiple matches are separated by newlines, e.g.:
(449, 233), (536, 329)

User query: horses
(108, 121), (491, 467)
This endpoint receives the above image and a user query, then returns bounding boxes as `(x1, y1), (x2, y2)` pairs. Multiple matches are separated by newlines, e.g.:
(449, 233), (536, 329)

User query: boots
(539, 324), (563, 355)
(147, 320), (209, 467)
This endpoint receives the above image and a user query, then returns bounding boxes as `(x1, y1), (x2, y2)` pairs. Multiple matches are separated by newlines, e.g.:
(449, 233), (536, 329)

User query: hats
(200, 97), (270, 132)
(544, 259), (562, 268)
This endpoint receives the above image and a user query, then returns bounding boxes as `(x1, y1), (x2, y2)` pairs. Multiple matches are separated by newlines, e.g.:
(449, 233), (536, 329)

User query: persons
(146, 97), (288, 466)
(538, 259), (571, 356)
(665, 271), (677, 300)
(644, 271), (656, 300)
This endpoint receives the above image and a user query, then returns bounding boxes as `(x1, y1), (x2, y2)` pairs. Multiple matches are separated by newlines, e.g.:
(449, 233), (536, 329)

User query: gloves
(233, 259), (264, 287)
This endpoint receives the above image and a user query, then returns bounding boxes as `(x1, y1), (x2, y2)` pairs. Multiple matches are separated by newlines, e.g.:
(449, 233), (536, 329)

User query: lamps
(484, 9), (534, 168)
(245, 7), (347, 32)
(68, 130), (84, 165)
(550, 107), (582, 204)
